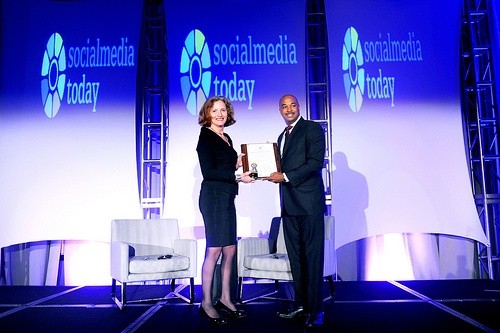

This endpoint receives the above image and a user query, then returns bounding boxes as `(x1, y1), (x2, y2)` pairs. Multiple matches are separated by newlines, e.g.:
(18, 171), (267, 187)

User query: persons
(196, 95), (256, 327)
(270, 95), (324, 329)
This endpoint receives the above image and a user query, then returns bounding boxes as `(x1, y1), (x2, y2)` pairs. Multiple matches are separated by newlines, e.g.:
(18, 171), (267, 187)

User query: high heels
(199, 303), (226, 323)
(216, 300), (246, 317)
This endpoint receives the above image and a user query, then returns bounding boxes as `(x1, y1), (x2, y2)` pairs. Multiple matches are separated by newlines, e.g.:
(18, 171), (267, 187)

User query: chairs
(236, 215), (336, 306)
(111, 219), (198, 311)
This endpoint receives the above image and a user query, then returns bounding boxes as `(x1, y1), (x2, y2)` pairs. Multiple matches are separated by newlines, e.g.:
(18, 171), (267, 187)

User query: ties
(285, 125), (292, 142)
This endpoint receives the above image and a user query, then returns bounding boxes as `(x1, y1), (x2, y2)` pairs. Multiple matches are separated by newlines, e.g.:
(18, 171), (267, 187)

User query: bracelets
(236, 175), (241, 182)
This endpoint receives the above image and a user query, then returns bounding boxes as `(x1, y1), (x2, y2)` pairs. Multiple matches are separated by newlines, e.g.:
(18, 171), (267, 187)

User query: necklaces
(209, 126), (229, 146)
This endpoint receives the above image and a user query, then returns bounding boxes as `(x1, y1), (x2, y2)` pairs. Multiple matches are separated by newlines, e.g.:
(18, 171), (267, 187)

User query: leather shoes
(306, 311), (325, 328)
(279, 308), (306, 319)
